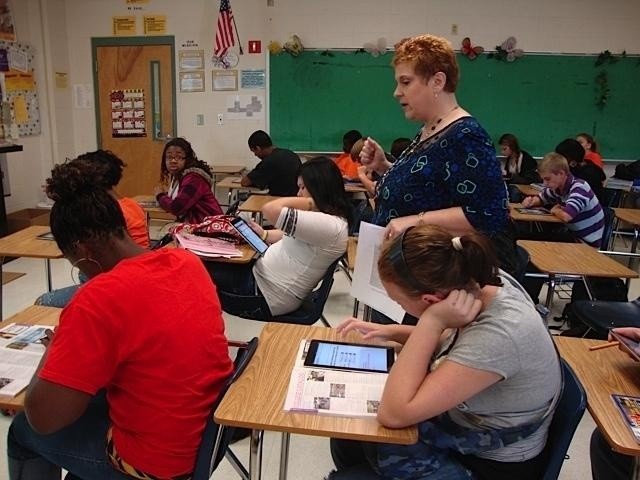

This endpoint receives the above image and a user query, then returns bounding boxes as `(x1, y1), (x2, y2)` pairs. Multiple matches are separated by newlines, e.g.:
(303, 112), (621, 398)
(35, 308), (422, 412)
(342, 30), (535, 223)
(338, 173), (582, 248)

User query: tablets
(304, 339), (394, 373)
(36, 232), (56, 240)
(229, 217), (269, 255)
(514, 207), (552, 215)
(608, 328), (640, 362)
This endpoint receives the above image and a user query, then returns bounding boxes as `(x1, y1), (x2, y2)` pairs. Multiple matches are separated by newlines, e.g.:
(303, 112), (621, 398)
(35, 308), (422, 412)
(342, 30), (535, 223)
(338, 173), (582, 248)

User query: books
(350, 220), (407, 325)
(1, 321), (58, 398)
(610, 394), (640, 445)
(282, 339), (398, 419)
(172, 233), (244, 260)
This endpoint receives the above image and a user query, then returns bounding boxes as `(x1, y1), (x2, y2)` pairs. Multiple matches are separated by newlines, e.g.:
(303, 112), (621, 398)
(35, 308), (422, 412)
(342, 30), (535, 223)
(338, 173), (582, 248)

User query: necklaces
(424, 105), (460, 136)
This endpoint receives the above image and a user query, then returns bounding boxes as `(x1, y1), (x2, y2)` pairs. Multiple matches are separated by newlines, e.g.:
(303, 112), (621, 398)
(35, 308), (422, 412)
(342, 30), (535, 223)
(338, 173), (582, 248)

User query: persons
(7, 158), (237, 480)
(336, 132), (640, 317)
(321, 223), (565, 479)
(241, 130), (303, 196)
(112, 96), (145, 129)
(589, 327), (639, 480)
(154, 136), (224, 224)
(202, 154), (357, 320)
(34, 148), (150, 309)
(352, 33), (517, 324)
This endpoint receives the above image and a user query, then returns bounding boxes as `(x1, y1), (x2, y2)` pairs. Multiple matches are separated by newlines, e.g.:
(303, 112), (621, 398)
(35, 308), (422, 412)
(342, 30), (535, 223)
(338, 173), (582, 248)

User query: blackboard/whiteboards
(266, 48), (640, 162)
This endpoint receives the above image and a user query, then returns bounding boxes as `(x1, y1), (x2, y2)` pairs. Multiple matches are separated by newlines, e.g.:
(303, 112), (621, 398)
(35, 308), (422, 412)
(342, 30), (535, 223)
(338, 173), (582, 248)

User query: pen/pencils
(589, 341), (619, 350)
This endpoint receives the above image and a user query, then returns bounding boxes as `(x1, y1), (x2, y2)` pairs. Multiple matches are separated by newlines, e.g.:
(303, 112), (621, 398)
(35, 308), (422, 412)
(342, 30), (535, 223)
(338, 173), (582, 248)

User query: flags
(213, 0), (234, 61)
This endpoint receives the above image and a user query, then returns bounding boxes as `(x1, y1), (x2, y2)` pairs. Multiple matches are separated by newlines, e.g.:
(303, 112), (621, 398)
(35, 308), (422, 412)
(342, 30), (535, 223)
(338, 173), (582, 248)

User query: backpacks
(572, 267), (630, 303)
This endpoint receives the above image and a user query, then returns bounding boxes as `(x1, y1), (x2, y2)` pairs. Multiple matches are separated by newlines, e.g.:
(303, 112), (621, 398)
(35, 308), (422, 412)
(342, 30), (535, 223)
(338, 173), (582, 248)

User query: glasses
(164, 153), (187, 160)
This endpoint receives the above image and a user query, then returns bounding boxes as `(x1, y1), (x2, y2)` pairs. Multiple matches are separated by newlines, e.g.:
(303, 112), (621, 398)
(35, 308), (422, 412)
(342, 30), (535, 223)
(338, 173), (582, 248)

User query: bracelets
(262, 229), (269, 242)
(307, 197), (313, 211)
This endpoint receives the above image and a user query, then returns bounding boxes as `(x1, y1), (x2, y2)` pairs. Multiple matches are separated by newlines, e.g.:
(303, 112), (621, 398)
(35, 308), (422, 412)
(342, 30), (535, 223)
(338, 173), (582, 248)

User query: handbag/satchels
(417, 417), (505, 461)
(169, 214), (246, 243)
